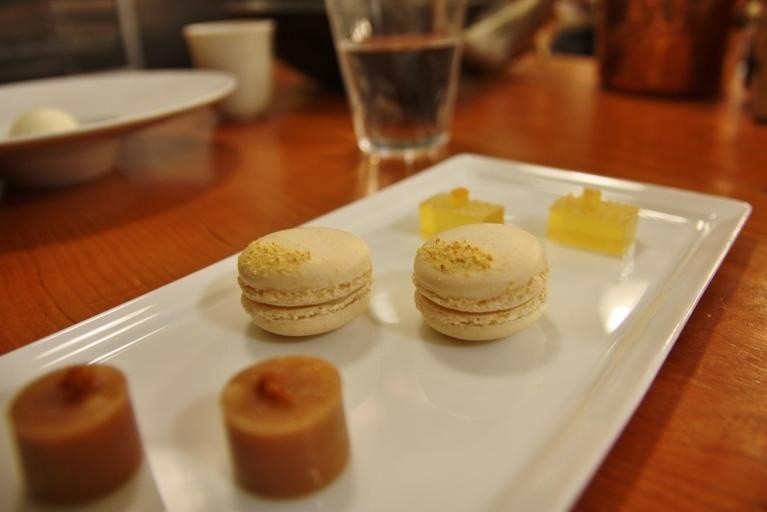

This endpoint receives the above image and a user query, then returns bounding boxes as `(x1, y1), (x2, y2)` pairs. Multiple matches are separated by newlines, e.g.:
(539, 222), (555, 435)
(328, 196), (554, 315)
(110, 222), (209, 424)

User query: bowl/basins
(0, 69), (246, 184)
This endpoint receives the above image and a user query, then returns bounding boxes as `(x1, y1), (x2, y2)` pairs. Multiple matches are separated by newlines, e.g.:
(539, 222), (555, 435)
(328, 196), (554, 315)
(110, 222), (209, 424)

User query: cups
(327, 0), (466, 158)
(180, 21), (276, 118)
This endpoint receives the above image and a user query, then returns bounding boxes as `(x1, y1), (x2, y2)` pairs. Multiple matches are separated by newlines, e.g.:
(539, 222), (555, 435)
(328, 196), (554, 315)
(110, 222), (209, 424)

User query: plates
(2, 148), (754, 508)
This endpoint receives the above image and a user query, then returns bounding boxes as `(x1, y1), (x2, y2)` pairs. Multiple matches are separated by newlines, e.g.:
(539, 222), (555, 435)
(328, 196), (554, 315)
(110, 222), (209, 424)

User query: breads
(238, 226), (373, 336)
(411, 224), (549, 341)
